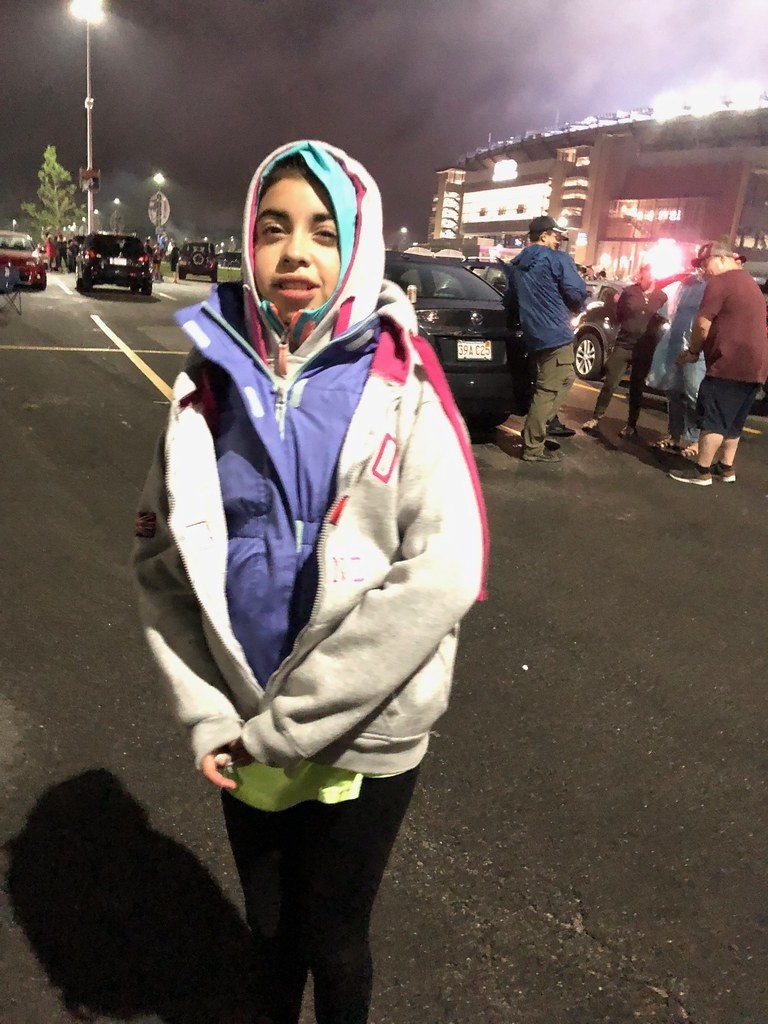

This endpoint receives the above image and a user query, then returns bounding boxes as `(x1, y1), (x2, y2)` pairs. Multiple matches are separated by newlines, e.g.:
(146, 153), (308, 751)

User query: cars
(0, 231), (48, 290)
(433, 255), (517, 330)
(568, 272), (768, 396)
(384, 250), (537, 431)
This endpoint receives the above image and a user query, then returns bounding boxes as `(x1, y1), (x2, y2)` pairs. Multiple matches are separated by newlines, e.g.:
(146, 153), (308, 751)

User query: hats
(529, 216), (568, 233)
(556, 233), (569, 242)
(732, 253), (747, 264)
(691, 243), (733, 268)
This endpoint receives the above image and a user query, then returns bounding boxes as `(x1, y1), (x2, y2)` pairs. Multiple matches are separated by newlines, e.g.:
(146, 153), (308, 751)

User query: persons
(44, 233), (56, 272)
(582, 265), (668, 437)
(585, 266), (607, 281)
(67, 240), (79, 273)
(507, 216), (577, 462)
(130, 138), (492, 1024)
(651, 241), (706, 457)
(145, 239), (153, 256)
(54, 232), (67, 274)
(151, 243), (165, 282)
(668, 239), (768, 486)
(168, 242), (179, 284)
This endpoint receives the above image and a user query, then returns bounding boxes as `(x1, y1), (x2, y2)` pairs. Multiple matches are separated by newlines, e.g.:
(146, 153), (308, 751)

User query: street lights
(113, 197), (121, 234)
(153, 171), (166, 244)
(68, 0), (109, 240)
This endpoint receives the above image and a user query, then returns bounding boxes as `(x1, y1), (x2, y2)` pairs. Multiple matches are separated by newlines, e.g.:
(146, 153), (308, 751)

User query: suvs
(176, 242), (219, 283)
(76, 233), (155, 296)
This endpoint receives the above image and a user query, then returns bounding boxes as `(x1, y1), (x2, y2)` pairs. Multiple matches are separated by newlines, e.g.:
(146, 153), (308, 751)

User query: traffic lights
(85, 171), (101, 193)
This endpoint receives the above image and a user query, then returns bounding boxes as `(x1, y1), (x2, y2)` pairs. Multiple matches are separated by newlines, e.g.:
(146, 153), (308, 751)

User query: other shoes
(617, 425), (637, 438)
(524, 450), (561, 462)
(653, 435), (678, 448)
(544, 435), (560, 451)
(680, 442), (700, 457)
(545, 424), (576, 437)
(669, 462), (713, 486)
(219, 988), (299, 1024)
(708, 460), (737, 482)
(581, 418), (599, 429)
